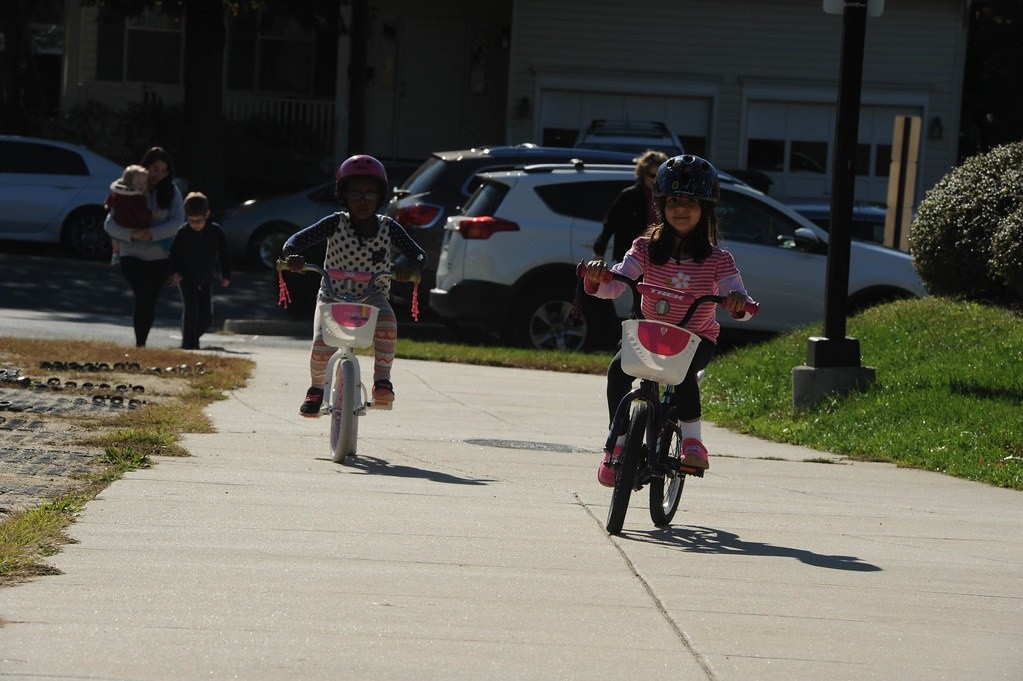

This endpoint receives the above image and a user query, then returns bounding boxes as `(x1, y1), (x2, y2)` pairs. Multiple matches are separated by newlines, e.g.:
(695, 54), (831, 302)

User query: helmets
(335, 154), (388, 200)
(651, 154), (720, 201)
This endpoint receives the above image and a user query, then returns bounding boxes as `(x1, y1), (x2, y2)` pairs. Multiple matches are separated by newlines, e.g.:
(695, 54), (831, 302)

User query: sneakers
(299, 385), (324, 415)
(597, 445), (625, 486)
(372, 379), (395, 410)
(678, 438), (709, 473)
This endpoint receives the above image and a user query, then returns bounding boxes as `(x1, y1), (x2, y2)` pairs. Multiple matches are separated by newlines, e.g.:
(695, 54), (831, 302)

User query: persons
(583, 153), (757, 488)
(103, 146), (185, 348)
(282, 155), (429, 418)
(593, 150), (668, 356)
(167, 191), (231, 350)
(105, 165), (155, 271)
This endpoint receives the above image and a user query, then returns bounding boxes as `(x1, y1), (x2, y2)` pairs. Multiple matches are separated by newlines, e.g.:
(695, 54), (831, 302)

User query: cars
(0, 134), (127, 262)
(717, 203), (917, 248)
(218, 175), (402, 272)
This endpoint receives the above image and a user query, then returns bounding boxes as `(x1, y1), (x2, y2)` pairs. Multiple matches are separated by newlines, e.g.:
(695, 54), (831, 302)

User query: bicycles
(275, 258), (420, 463)
(570, 260), (759, 535)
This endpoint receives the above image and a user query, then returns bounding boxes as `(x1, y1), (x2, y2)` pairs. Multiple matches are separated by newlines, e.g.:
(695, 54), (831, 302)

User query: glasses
(187, 216), (206, 224)
(646, 169), (657, 178)
(341, 191), (384, 200)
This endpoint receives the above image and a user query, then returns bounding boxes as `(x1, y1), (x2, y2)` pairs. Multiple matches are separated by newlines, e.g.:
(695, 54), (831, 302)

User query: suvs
(384, 143), (750, 311)
(425, 160), (933, 353)
(573, 119), (685, 162)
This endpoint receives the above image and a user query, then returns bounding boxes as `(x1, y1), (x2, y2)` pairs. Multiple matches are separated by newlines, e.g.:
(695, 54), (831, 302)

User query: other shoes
(150, 246), (168, 258)
(111, 254), (120, 266)
